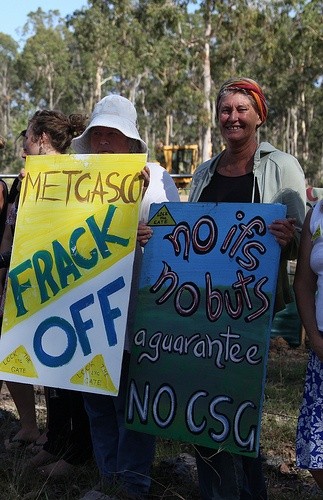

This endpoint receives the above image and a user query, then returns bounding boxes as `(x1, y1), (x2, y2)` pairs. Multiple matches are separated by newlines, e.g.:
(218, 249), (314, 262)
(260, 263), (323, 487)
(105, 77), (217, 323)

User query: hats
(69, 95), (149, 154)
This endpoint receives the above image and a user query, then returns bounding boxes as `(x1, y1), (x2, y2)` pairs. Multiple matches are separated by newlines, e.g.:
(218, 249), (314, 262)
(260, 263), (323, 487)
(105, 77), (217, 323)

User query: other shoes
(22, 452), (57, 471)
(36, 459), (88, 481)
(79, 489), (118, 500)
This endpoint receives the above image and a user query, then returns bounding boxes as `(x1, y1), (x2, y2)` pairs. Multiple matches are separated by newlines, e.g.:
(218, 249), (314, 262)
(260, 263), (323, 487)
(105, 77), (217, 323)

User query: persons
(68, 93), (180, 500)
(137, 78), (306, 500)
(292, 195), (323, 490)
(0, 109), (92, 481)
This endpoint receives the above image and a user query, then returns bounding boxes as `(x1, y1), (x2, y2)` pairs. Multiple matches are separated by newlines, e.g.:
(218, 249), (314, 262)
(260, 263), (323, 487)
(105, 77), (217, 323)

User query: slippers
(0, 432), (33, 455)
(20, 440), (44, 457)
